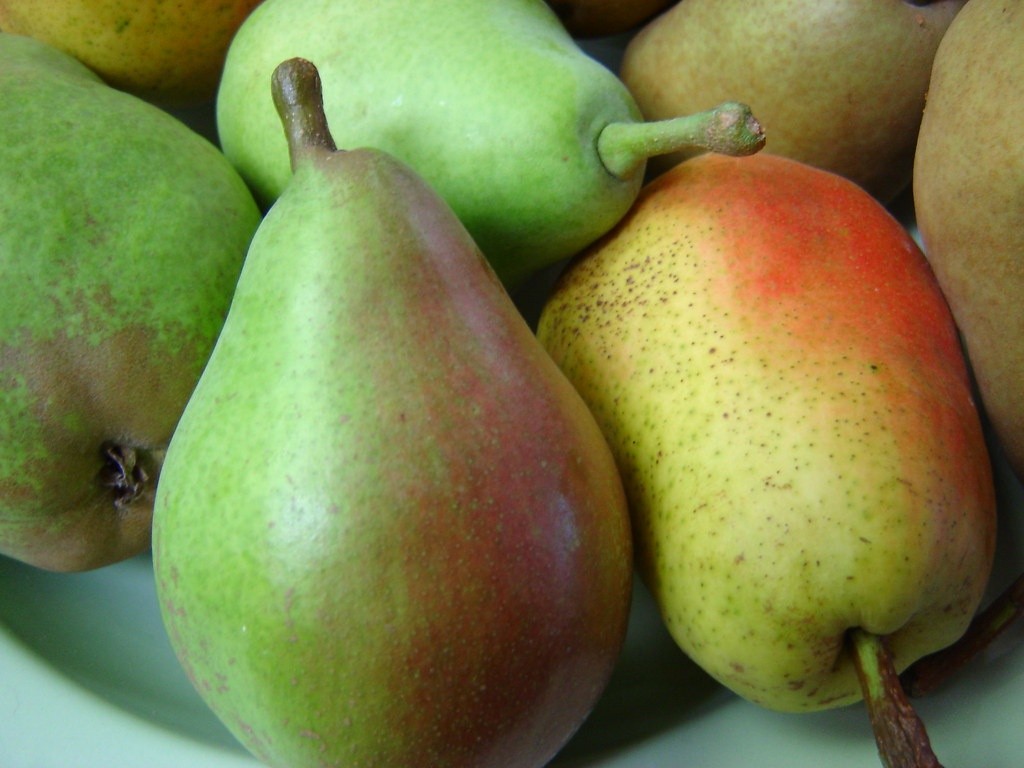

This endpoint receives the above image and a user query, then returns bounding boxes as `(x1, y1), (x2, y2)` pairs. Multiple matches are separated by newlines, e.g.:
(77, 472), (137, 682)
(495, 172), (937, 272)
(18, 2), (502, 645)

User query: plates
(0, 0), (1024, 768)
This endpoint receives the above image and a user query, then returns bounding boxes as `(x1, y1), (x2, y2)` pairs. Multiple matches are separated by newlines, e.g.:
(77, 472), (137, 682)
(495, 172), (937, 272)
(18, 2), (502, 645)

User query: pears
(0, 0), (1024, 768)
